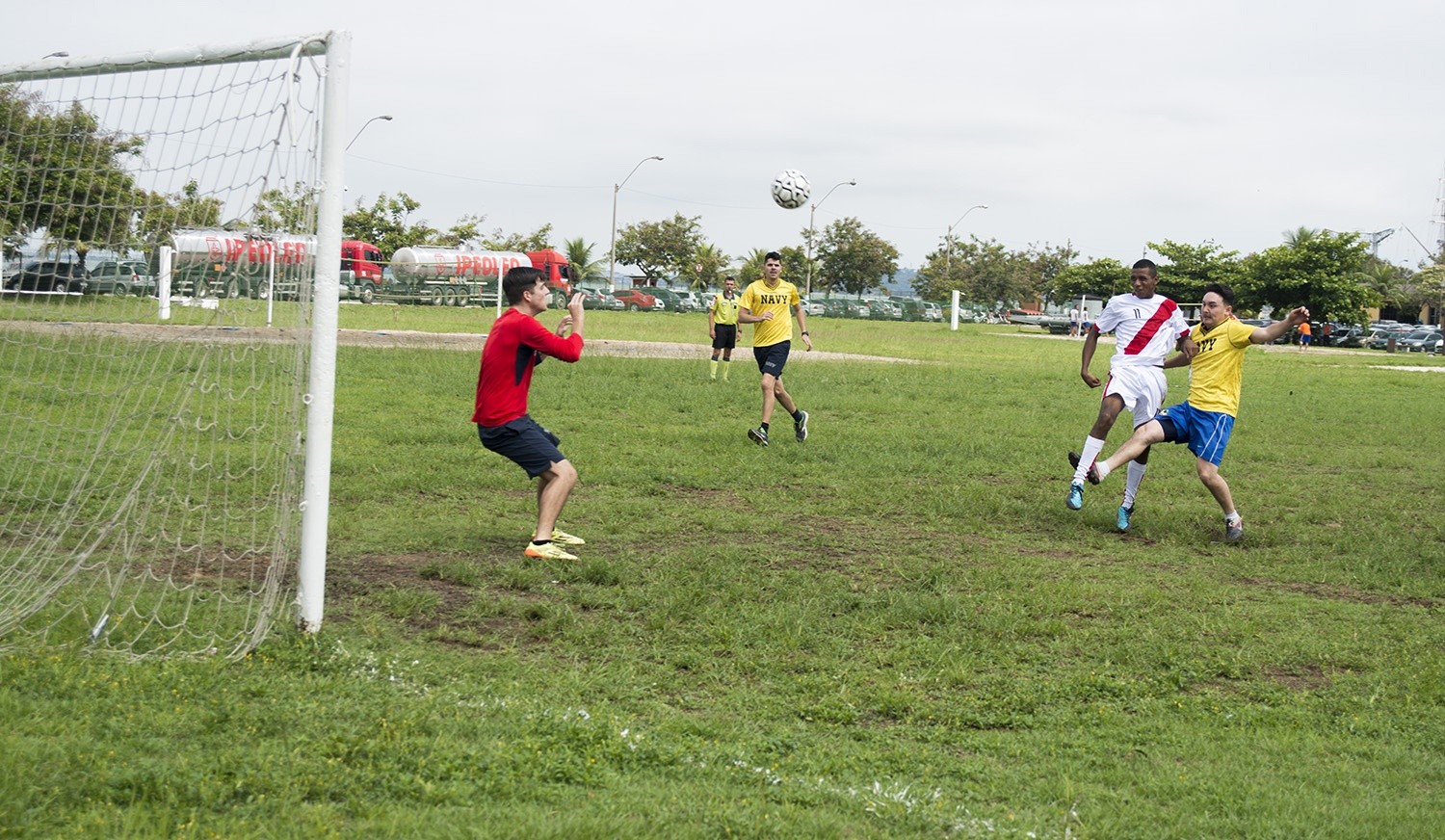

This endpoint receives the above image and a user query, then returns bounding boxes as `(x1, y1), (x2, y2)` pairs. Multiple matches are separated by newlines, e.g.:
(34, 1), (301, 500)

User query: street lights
(606, 155), (663, 293)
(943, 204), (990, 276)
(804, 180), (856, 296)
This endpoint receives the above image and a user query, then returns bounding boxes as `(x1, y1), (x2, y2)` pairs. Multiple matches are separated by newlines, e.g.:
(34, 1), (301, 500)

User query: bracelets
(801, 331), (810, 335)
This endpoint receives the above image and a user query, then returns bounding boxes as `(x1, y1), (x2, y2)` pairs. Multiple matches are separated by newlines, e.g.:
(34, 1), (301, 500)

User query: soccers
(772, 168), (810, 208)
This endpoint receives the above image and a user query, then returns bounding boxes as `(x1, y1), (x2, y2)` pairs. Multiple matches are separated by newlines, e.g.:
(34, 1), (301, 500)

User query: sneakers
(1068, 450), (1100, 484)
(525, 540), (580, 561)
(722, 376), (730, 384)
(710, 374), (716, 382)
(1116, 502), (1135, 532)
(794, 411), (809, 441)
(747, 427), (768, 446)
(529, 527), (586, 544)
(1224, 518), (1244, 540)
(1065, 479), (1084, 509)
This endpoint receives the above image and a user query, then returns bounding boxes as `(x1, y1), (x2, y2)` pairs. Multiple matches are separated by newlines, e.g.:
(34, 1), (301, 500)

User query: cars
(791, 296), (946, 321)
(95, 259), (150, 269)
(956, 305), (1047, 325)
(1234, 316), (1444, 355)
(580, 285), (717, 315)
(2, 261), (29, 286)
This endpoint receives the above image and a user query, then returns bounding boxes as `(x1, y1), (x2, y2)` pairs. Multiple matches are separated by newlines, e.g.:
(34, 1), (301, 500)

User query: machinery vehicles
(389, 245), (576, 309)
(162, 229), (386, 302)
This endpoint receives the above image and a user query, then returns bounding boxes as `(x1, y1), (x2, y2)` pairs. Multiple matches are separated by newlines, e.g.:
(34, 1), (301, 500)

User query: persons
(1066, 258), (1203, 536)
(737, 250), (814, 448)
(1298, 320), (1312, 351)
(709, 276), (740, 384)
(1070, 286), (1312, 544)
(470, 266), (587, 560)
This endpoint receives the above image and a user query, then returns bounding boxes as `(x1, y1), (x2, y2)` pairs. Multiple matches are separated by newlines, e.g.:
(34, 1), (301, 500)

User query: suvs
(5, 263), (88, 294)
(82, 264), (155, 298)
